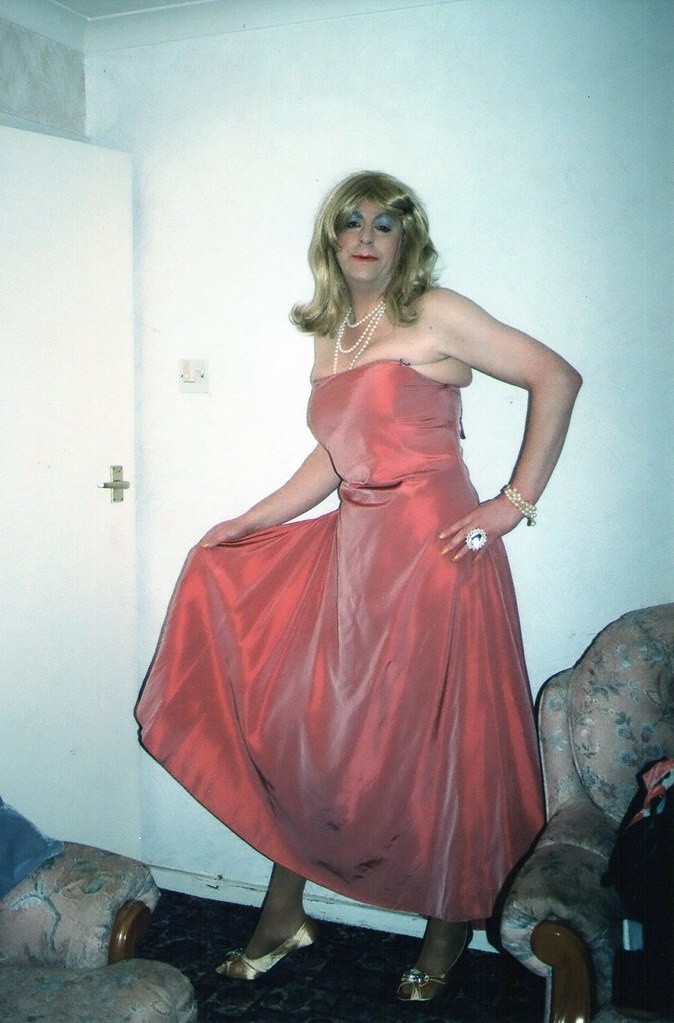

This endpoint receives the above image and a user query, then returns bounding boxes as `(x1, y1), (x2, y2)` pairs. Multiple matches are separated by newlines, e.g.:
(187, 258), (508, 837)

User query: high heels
(215, 914), (318, 980)
(396, 919), (473, 1000)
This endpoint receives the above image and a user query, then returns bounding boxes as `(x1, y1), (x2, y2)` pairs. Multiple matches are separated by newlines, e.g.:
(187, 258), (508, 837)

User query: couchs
(0, 841), (200, 1023)
(499, 602), (674, 1023)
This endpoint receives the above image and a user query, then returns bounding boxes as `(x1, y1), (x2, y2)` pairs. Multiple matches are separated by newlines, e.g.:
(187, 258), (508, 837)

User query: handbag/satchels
(600, 756), (674, 1015)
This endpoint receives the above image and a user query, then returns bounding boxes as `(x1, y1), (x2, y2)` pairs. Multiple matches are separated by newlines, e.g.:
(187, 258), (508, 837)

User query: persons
(134, 170), (582, 1002)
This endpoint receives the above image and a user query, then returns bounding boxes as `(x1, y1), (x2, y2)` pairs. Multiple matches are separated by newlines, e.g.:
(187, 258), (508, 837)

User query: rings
(466, 529), (487, 550)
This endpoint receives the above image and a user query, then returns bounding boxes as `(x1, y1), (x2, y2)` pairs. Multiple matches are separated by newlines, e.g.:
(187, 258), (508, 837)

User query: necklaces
(333, 298), (386, 375)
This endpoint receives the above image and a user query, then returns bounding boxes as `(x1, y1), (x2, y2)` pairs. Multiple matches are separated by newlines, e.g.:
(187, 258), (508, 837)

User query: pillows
(0, 796), (65, 902)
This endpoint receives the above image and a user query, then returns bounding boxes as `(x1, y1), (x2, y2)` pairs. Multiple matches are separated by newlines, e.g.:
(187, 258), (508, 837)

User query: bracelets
(504, 485), (537, 526)
